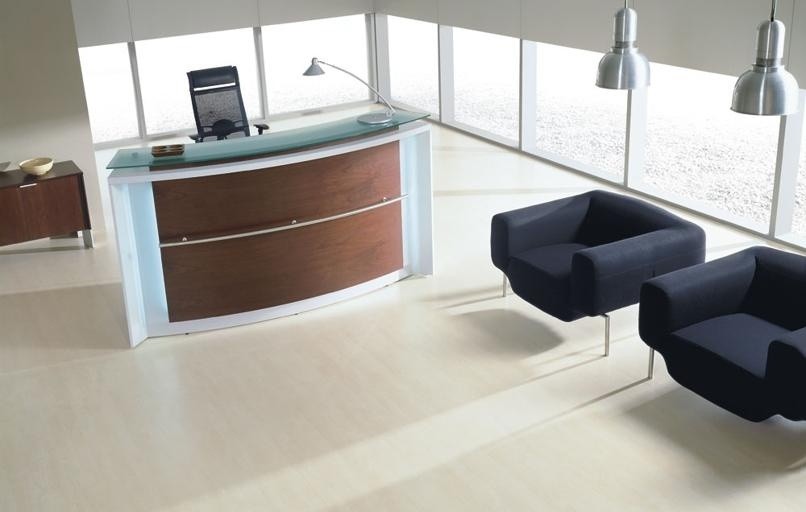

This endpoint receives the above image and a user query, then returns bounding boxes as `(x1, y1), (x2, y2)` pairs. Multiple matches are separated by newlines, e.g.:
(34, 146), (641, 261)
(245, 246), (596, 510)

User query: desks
(0, 160), (94, 249)
(106, 109), (434, 348)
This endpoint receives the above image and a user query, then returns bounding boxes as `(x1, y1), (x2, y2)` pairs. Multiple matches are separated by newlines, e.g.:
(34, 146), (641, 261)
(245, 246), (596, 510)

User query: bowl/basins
(19, 157), (53, 176)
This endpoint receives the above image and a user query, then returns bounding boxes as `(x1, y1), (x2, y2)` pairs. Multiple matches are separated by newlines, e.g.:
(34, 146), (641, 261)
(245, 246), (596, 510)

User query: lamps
(730, 0), (800, 116)
(303, 57), (396, 125)
(595, 0), (651, 90)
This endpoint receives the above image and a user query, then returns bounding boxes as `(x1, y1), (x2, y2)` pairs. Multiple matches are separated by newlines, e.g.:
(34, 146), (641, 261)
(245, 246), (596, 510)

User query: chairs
(491, 190), (706, 357)
(638, 246), (806, 423)
(186, 65), (269, 143)
